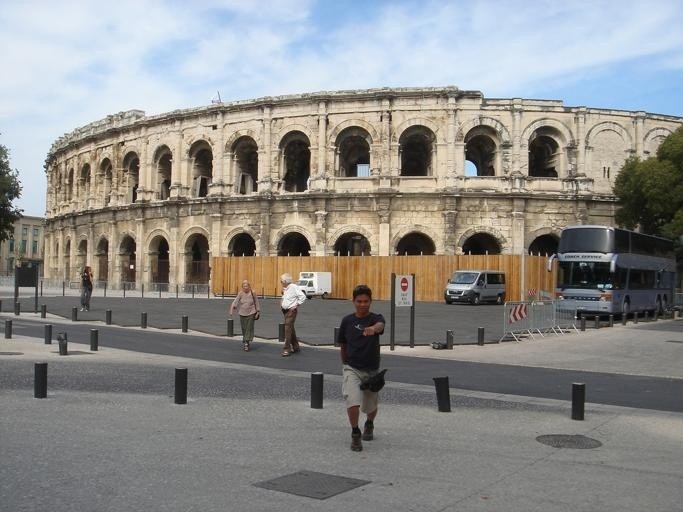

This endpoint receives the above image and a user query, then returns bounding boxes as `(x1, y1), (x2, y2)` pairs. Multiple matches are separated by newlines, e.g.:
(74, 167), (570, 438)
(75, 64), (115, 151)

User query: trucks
(294, 271), (333, 300)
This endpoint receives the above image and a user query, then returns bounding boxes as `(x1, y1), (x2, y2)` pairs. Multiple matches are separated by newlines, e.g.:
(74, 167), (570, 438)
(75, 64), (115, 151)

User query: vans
(443, 268), (508, 307)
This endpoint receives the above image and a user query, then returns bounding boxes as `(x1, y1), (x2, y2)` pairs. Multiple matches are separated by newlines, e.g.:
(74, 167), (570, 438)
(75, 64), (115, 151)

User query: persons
(338, 285), (385, 451)
(280, 273), (306, 356)
(230, 280), (261, 350)
(79, 266), (93, 312)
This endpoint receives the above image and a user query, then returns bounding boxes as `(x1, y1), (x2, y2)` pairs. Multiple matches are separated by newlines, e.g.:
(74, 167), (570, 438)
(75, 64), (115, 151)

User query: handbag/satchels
(253, 311), (259, 320)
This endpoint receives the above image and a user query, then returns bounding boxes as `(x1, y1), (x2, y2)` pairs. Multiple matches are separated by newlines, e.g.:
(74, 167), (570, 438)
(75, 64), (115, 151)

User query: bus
(546, 224), (678, 322)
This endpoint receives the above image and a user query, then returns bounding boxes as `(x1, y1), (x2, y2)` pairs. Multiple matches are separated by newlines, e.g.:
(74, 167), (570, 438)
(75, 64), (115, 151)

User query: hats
(359, 367), (388, 393)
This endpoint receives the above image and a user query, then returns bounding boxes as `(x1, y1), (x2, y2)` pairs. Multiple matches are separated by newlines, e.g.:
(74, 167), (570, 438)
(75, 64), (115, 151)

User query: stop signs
(400, 277), (408, 291)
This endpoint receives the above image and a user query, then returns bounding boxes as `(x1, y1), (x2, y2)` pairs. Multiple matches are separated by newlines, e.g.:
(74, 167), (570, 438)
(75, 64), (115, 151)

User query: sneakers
(242, 341), (249, 352)
(349, 430), (363, 451)
(363, 419), (373, 441)
(281, 345), (299, 356)
